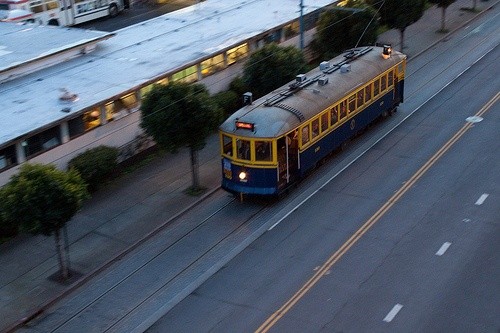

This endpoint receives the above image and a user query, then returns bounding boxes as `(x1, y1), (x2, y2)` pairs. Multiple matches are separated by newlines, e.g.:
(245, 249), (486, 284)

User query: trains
(220, 45), (408, 206)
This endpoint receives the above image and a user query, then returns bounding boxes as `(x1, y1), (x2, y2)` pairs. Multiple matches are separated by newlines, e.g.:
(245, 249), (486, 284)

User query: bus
(0, 0), (125, 27)
